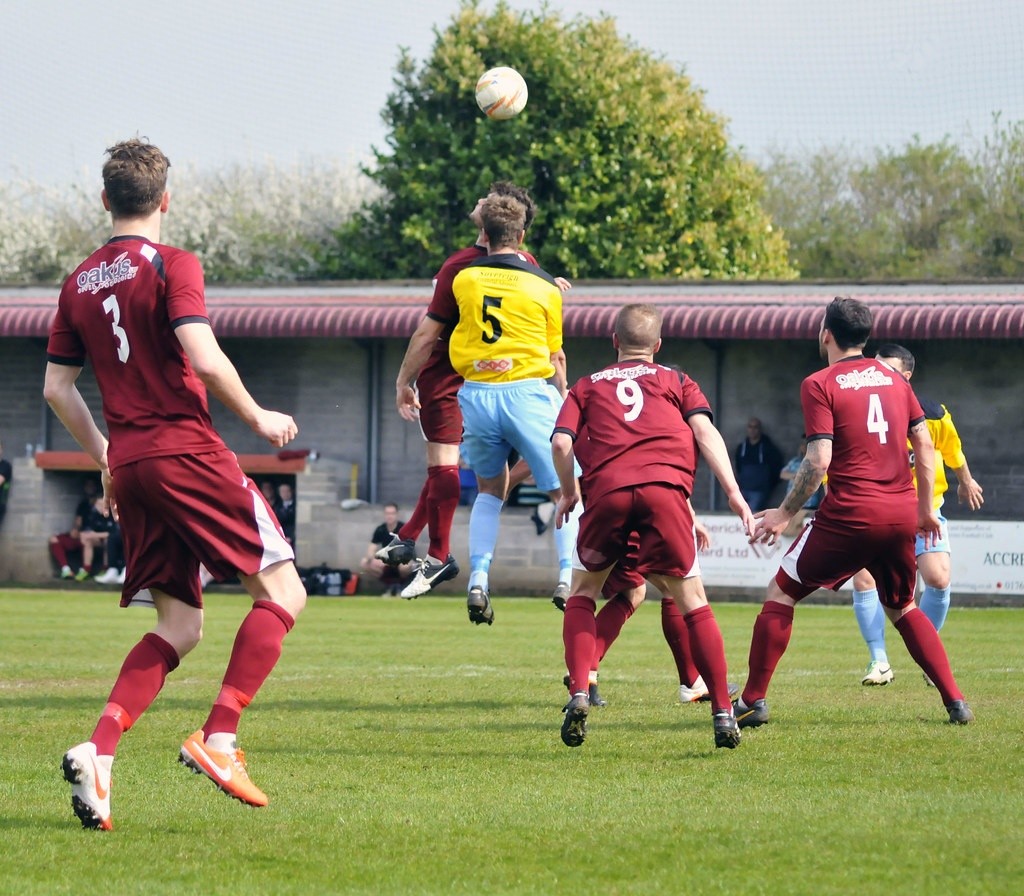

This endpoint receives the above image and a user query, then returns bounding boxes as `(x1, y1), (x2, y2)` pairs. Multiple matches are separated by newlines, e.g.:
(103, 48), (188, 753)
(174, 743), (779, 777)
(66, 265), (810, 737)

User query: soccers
(475, 67), (528, 120)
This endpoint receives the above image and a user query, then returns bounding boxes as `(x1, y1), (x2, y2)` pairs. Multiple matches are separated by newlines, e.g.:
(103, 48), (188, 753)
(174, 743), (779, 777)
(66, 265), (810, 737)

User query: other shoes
(382, 587), (397, 598)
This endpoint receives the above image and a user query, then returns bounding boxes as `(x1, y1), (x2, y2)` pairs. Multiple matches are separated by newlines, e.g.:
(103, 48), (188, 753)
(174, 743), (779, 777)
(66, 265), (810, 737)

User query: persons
(373, 175), (540, 599)
(735, 418), (826, 509)
(551, 303), (759, 749)
(50, 483), (295, 589)
(448, 196), (586, 626)
(42, 128), (300, 831)
(564, 363), (740, 705)
(732, 296), (974, 727)
(0, 446), (13, 526)
(362, 502), (419, 599)
(821, 345), (984, 686)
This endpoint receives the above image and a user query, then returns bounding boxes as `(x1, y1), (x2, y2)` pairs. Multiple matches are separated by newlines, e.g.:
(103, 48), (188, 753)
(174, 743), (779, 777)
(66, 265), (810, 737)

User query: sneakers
(401, 554), (459, 599)
(561, 696), (590, 747)
(563, 675), (607, 707)
(178, 728), (267, 808)
(947, 700), (975, 726)
(733, 695), (769, 730)
(712, 708), (742, 748)
(93, 567), (126, 585)
(552, 584), (569, 612)
(923, 672), (936, 687)
(679, 674), (739, 703)
(467, 584), (494, 626)
(862, 661), (895, 685)
(61, 566), (89, 582)
(61, 741), (115, 832)
(374, 532), (416, 567)
